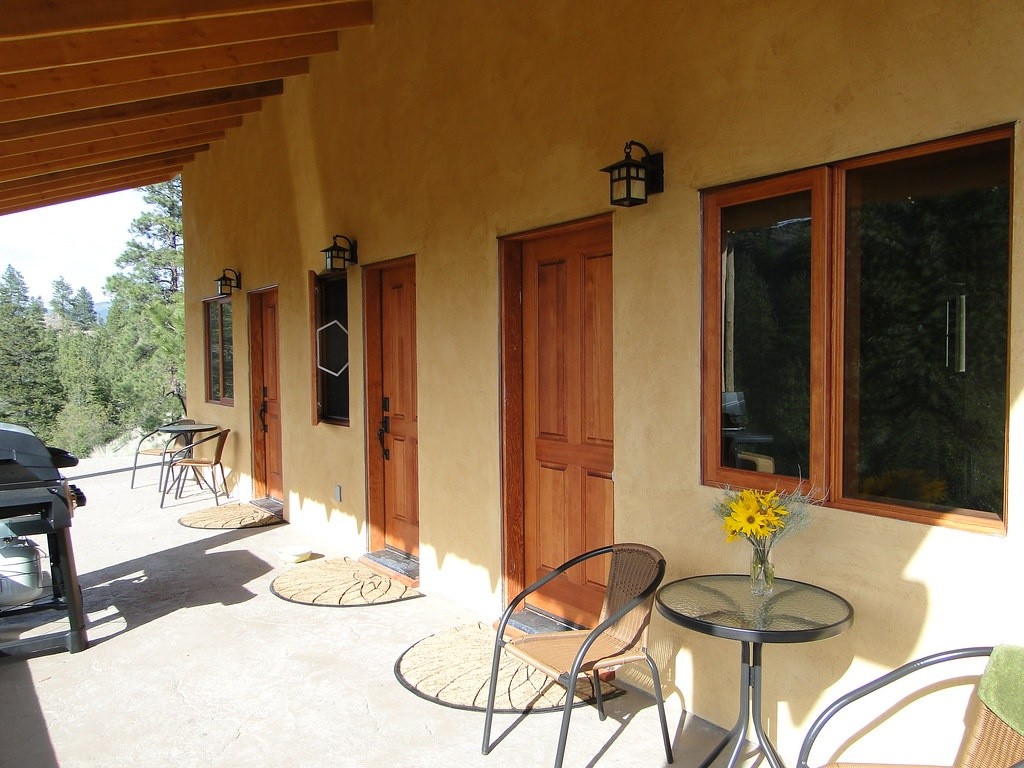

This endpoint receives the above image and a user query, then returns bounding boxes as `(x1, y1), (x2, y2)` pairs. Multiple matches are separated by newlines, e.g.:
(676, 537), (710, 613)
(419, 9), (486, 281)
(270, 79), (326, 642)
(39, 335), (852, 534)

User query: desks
(656, 574), (854, 768)
(160, 424), (218, 497)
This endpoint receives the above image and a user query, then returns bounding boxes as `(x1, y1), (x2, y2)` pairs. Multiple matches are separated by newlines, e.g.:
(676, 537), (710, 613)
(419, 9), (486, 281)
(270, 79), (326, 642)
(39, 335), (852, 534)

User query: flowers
(711, 465), (832, 585)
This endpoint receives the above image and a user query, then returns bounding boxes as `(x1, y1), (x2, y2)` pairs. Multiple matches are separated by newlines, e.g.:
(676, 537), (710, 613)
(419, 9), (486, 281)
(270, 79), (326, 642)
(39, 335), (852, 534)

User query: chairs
(160, 429), (231, 508)
(482, 543), (674, 768)
(131, 419), (195, 492)
(796, 647), (1024, 768)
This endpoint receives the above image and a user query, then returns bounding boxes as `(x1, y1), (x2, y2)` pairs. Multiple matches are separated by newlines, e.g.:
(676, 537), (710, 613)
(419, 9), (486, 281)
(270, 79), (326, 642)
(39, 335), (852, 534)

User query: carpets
(270, 556), (425, 607)
(179, 500), (287, 529)
(394, 620), (626, 714)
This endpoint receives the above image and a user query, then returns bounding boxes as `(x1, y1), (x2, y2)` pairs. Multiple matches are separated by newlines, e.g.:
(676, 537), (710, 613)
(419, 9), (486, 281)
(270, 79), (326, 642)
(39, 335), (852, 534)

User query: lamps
(320, 235), (358, 274)
(598, 140), (664, 207)
(214, 268), (241, 297)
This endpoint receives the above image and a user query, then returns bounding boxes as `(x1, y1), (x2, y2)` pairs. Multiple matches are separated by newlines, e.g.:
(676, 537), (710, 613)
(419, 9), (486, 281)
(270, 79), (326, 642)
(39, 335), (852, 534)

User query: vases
(751, 547), (775, 594)
